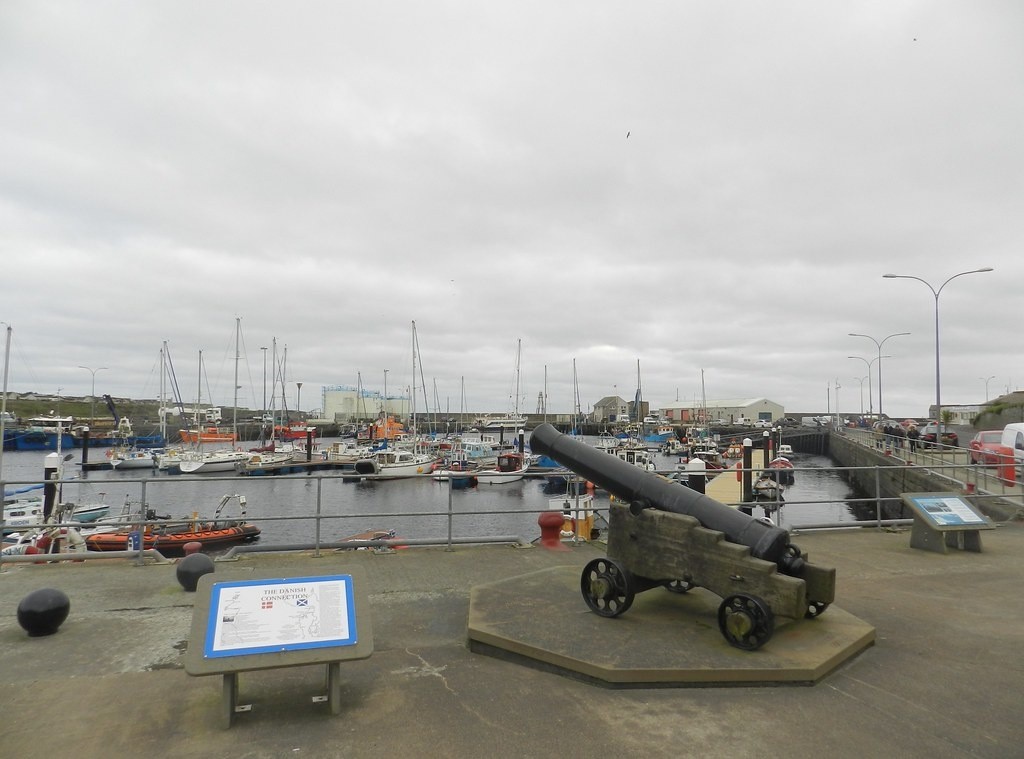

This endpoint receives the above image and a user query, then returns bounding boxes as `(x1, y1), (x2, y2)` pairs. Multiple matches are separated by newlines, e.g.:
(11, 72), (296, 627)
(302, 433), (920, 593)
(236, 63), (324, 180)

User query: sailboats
(334, 321), (543, 484)
(110, 318), (331, 474)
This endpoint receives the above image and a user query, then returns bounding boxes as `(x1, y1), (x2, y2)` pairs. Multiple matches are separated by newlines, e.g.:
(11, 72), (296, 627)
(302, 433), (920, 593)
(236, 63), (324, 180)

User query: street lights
(848, 355), (891, 426)
(848, 333), (910, 431)
(79, 365), (108, 427)
(882, 267), (994, 452)
(979, 376), (996, 403)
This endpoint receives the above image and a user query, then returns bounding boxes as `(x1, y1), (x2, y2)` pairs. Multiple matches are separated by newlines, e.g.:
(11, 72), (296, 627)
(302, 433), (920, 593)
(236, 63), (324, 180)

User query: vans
(802, 417), (829, 427)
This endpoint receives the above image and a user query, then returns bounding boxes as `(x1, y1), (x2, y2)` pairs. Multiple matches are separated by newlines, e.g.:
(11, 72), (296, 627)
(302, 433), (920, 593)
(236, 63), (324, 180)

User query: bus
(26, 418), (73, 433)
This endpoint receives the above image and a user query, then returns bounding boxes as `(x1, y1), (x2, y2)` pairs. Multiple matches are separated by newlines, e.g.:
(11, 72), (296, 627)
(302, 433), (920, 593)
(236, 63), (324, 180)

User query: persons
(891, 425), (903, 456)
(876, 423), (893, 450)
(909, 429), (917, 453)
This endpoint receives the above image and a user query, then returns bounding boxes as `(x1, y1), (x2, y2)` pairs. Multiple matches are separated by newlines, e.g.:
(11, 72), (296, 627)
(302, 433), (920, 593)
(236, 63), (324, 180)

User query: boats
(616, 428), (743, 469)
(2, 498), (261, 552)
(777, 445), (794, 459)
(757, 495), (786, 512)
(756, 457), (794, 498)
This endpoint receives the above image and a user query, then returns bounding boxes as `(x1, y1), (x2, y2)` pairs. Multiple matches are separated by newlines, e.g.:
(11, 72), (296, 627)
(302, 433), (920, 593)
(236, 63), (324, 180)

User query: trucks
(253, 414), (273, 422)
(617, 415), (630, 424)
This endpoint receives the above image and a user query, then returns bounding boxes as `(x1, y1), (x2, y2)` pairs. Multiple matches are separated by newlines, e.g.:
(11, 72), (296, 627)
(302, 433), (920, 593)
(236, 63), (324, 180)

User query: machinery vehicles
(158, 407), (222, 425)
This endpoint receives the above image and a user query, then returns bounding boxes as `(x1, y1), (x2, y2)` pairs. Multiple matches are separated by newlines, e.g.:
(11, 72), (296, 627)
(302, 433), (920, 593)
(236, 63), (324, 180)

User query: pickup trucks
(900, 420), (919, 425)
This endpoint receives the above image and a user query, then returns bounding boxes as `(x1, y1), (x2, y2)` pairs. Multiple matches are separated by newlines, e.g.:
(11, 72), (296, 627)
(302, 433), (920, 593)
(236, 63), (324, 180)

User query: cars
(860, 418), (871, 427)
(968, 431), (1004, 468)
(917, 426), (959, 449)
(409, 415), (457, 422)
(708, 417), (799, 429)
(873, 420), (906, 432)
(1002, 423), (1024, 477)
